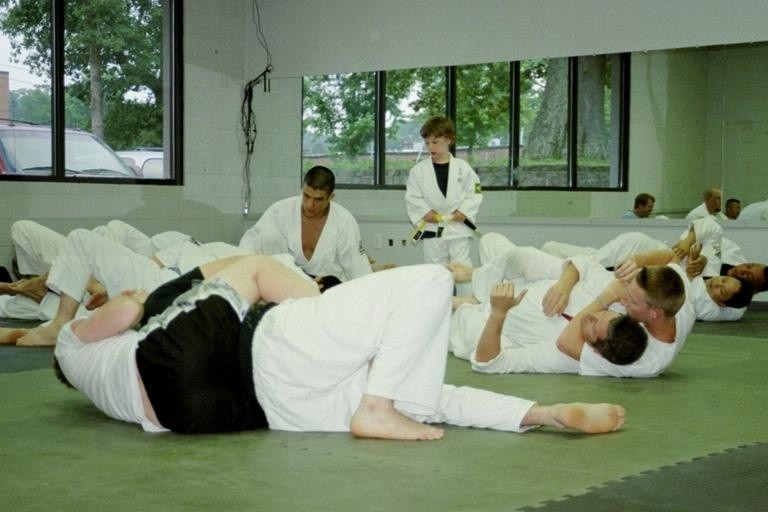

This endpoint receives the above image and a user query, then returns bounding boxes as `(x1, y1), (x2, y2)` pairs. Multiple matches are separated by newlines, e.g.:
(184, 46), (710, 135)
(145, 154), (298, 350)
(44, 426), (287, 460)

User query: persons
(618, 191), (657, 220)
(445, 219), (767, 377)
(685, 187), (723, 220)
(0, 215), (628, 442)
(240, 164), (371, 278)
(401, 117), (483, 299)
(725, 197), (742, 216)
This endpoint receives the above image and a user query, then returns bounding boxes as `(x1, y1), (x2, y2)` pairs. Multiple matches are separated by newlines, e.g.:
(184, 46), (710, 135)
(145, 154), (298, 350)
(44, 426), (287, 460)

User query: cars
(0, 117), (164, 177)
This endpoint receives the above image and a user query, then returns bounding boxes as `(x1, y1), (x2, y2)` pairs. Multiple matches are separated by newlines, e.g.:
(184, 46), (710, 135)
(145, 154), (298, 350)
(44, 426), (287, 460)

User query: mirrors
(299, 41), (768, 225)
